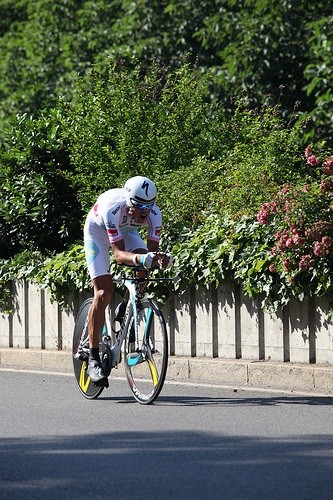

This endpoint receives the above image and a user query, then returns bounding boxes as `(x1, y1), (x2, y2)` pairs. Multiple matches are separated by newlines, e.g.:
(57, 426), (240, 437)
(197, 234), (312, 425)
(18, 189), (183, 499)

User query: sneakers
(87, 358), (106, 382)
(117, 312), (135, 341)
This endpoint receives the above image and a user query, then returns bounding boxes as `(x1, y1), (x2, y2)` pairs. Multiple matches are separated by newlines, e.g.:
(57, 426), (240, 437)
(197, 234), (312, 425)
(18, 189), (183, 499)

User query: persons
(84, 176), (173, 385)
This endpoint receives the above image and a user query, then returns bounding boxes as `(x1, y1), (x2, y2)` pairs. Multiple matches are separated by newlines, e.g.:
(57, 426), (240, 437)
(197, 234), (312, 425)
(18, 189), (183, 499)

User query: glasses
(137, 203), (154, 208)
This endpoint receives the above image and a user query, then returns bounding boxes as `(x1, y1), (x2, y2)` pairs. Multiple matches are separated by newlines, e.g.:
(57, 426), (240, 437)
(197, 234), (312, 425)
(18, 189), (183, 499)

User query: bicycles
(72, 261), (180, 406)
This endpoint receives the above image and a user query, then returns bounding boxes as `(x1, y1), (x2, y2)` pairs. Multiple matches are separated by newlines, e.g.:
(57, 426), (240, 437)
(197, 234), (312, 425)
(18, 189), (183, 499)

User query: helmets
(123, 176), (157, 208)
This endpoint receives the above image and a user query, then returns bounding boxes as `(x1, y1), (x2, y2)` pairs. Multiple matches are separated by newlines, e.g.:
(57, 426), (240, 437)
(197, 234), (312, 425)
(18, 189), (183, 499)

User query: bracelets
(140, 254), (144, 265)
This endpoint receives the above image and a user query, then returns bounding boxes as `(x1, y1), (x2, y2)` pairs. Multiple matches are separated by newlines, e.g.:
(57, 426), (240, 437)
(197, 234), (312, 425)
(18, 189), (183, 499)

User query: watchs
(132, 254), (141, 266)
